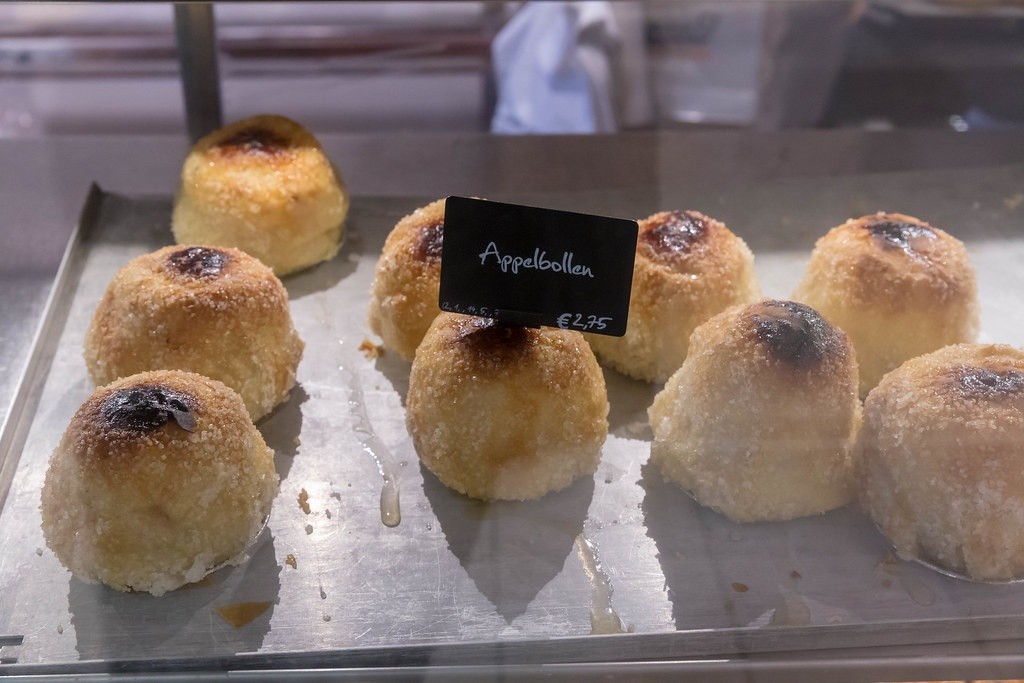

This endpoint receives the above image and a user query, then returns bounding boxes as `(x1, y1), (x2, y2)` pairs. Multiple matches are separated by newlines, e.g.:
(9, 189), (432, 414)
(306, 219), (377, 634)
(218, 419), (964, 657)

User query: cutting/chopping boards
(1, 180), (1024, 675)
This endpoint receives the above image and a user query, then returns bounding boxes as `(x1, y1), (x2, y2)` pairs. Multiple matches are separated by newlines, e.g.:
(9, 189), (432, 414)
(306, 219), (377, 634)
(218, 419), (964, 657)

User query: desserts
(39, 114), (1022, 597)
